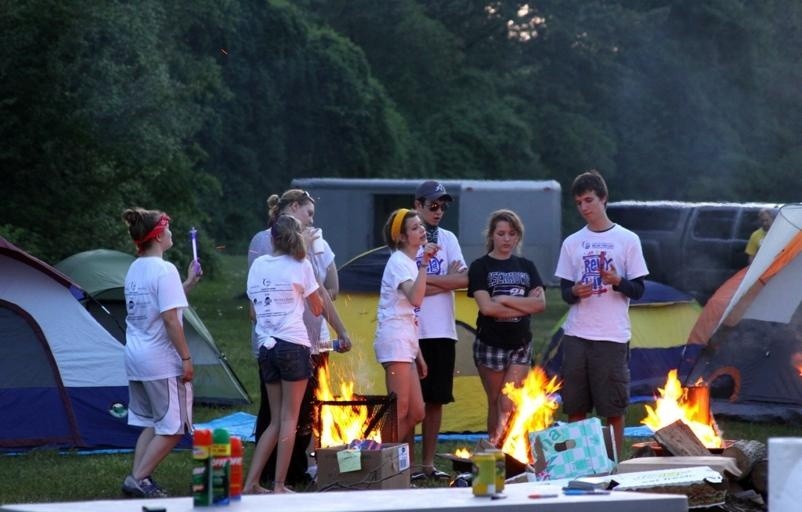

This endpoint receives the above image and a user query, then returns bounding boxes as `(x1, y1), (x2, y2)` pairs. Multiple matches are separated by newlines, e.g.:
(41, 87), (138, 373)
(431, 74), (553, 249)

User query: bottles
(191, 428), (242, 508)
(316, 337), (346, 352)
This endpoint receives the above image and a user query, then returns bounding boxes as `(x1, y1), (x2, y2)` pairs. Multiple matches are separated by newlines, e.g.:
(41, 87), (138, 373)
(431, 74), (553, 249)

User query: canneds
(484, 449), (505, 492)
(472, 452), (496, 496)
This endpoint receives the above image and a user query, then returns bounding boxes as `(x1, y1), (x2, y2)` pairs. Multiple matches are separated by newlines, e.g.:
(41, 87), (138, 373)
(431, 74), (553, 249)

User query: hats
(415, 180), (454, 202)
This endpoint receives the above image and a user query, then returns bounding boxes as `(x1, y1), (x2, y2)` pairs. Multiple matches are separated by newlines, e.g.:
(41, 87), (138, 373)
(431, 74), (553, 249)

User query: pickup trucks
(605, 199), (787, 307)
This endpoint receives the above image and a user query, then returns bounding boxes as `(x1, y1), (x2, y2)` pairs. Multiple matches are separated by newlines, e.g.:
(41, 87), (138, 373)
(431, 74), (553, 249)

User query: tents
(675, 200), (802, 420)
(53, 248), (254, 406)
(325, 245), (535, 440)
(0, 236), (194, 456)
(540, 279), (704, 406)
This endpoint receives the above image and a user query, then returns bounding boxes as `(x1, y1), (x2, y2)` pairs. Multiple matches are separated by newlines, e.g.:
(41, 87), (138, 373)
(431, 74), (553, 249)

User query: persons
(373, 208), (443, 444)
(240, 190), (352, 494)
(123, 207), (203, 499)
(467, 208), (545, 442)
(402, 181), (469, 484)
(743, 208), (774, 264)
(553, 170), (649, 463)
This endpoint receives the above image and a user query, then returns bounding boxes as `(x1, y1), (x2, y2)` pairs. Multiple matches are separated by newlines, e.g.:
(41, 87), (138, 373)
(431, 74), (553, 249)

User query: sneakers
(428, 469), (451, 481)
(410, 471), (427, 483)
(122, 474), (167, 499)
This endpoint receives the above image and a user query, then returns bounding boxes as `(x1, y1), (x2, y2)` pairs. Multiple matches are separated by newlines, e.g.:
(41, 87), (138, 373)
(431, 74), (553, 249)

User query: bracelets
(181, 357), (191, 361)
(420, 264), (429, 268)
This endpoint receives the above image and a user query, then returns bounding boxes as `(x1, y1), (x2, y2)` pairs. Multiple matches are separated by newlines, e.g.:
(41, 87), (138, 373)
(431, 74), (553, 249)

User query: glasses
(425, 203), (448, 211)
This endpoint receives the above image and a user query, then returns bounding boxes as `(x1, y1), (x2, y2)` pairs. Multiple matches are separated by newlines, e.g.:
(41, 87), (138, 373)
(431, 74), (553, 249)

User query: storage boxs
(529, 417), (621, 482)
(313, 440), (413, 490)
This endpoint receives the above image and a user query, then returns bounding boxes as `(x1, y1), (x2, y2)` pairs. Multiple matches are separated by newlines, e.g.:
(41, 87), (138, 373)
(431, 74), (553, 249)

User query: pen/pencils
(528, 494), (558, 498)
(562, 489), (611, 495)
